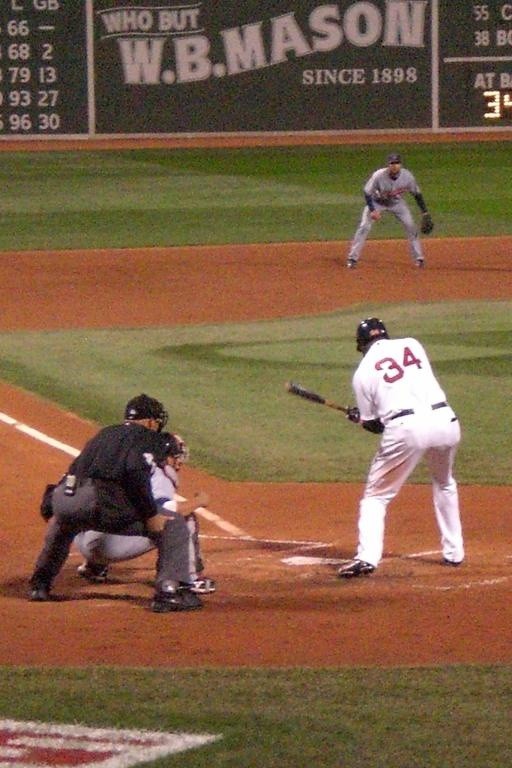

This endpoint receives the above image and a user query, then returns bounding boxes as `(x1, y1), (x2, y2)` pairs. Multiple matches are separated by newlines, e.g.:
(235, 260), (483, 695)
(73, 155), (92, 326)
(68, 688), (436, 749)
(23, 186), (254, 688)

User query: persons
(74, 433), (218, 596)
(28, 393), (205, 614)
(346, 154), (433, 270)
(336, 315), (465, 576)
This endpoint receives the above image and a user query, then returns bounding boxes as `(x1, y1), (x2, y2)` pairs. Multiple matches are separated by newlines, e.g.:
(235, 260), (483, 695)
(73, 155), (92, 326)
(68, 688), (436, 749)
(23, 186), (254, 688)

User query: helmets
(357, 318), (386, 350)
(126, 393), (188, 471)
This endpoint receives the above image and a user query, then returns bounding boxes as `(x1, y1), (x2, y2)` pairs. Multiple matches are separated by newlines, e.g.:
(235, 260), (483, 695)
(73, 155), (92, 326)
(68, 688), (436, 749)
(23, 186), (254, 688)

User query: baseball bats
(285, 384), (349, 416)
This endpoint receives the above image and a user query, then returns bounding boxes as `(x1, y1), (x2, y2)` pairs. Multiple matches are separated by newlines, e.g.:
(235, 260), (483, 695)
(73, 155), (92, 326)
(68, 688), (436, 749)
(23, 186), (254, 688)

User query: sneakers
(30, 588), (47, 600)
(337, 559), (374, 577)
(151, 577), (215, 612)
(78, 563), (107, 581)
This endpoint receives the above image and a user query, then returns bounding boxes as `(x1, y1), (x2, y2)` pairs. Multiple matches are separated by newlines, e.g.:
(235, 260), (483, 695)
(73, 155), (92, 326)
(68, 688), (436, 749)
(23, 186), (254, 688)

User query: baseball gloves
(421, 214), (435, 233)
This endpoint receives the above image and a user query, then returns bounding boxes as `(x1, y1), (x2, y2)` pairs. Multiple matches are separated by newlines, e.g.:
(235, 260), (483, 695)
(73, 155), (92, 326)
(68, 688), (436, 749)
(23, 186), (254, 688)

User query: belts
(392, 403), (446, 418)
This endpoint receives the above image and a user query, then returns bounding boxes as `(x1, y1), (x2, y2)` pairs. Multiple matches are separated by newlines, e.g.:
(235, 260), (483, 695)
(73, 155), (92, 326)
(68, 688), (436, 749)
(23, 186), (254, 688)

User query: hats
(389, 155), (401, 163)
